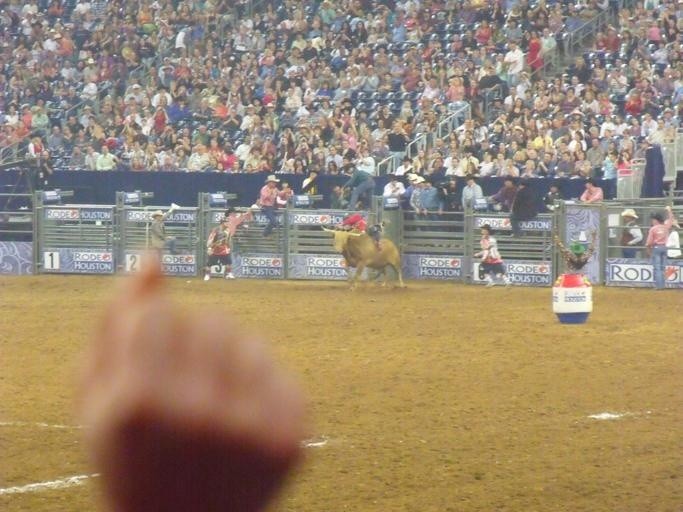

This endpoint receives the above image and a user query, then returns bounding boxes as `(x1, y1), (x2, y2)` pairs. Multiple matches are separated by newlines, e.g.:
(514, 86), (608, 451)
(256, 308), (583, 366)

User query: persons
(74, 250), (304, 512)
(0, 0), (682, 288)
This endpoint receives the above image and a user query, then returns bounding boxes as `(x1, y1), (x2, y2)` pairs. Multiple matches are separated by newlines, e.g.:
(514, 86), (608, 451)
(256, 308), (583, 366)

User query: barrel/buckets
(551, 273), (593, 324)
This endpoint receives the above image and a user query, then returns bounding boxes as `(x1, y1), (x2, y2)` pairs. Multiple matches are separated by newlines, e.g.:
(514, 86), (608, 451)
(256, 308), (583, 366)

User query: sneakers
(504, 280), (512, 288)
(204, 274), (210, 280)
(226, 273), (234, 279)
(486, 281), (494, 288)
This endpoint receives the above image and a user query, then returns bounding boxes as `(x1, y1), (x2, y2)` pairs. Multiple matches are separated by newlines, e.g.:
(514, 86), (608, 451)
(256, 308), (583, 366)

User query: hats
(621, 209), (639, 218)
(505, 175), (511, 181)
(464, 175), (477, 181)
(481, 225), (490, 229)
(85, 59), (97, 66)
(161, 66), (174, 73)
(225, 208), (235, 216)
(220, 219), (228, 224)
(265, 175), (280, 183)
(245, 103), (273, 109)
(571, 110), (586, 120)
(157, 85), (169, 91)
(152, 210), (163, 218)
(367, 64), (376, 71)
(667, 219), (680, 229)
(663, 108), (673, 116)
(549, 181), (559, 189)
(520, 180), (526, 185)
(399, 156), (425, 184)
(42, 21), (62, 40)
(514, 126), (524, 132)
(651, 212), (665, 221)
(341, 98), (353, 105)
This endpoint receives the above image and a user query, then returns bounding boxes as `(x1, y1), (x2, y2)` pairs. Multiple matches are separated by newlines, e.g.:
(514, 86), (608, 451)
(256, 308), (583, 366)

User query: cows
(320, 225), (405, 288)
